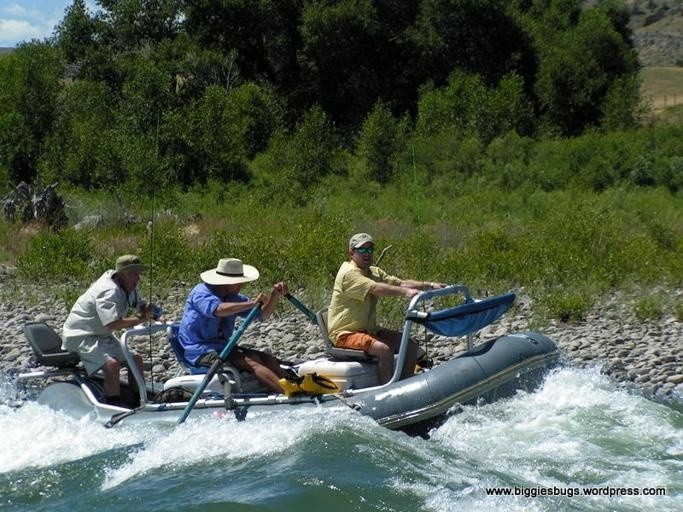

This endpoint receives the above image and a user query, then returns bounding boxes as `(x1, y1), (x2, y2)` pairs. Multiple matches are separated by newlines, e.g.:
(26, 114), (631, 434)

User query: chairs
(312, 298), (378, 364)
(19, 321), (82, 371)
(159, 322), (265, 402)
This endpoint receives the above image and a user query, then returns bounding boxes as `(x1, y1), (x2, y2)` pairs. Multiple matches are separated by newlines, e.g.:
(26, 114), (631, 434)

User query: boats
(21, 282), (562, 436)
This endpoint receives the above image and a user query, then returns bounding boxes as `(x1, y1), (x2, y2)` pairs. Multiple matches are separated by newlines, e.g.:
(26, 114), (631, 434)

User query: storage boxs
(297, 351), (404, 393)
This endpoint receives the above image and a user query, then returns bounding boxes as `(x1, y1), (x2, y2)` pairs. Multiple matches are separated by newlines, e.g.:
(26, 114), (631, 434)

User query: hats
(349, 233), (374, 251)
(110, 255), (149, 278)
(200, 258), (259, 286)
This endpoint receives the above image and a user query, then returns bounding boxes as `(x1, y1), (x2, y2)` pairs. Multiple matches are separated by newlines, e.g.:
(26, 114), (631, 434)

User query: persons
(177, 256), (290, 394)
(60, 252), (172, 405)
(326, 232), (449, 384)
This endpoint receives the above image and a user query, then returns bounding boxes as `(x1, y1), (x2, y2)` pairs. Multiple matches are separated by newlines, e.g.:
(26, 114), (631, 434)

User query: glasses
(353, 247), (372, 254)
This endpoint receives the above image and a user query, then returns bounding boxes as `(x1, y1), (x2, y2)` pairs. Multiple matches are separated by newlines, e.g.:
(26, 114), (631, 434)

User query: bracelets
(135, 311), (145, 321)
(429, 281), (435, 289)
